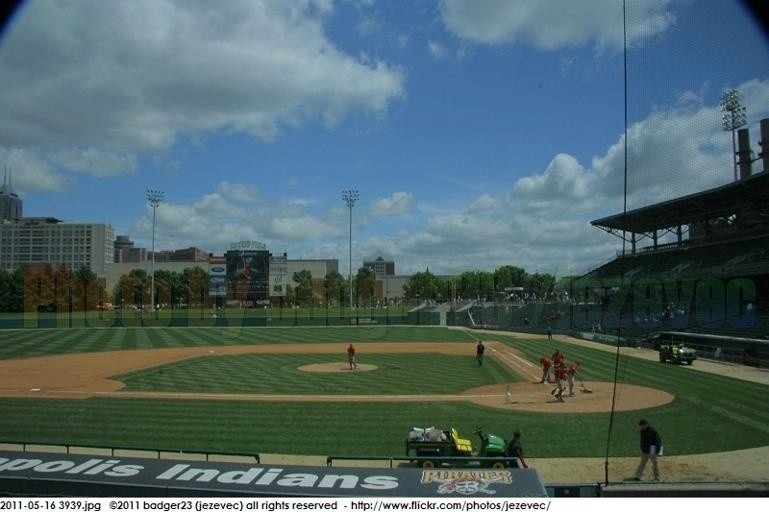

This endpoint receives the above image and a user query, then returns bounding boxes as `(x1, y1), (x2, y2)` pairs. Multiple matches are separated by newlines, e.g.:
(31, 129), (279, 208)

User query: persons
(118, 302), (169, 314)
(634, 295), (689, 325)
(507, 428), (528, 469)
(236, 250), (253, 280)
(747, 300), (753, 313)
(505, 385), (512, 405)
(347, 344), (359, 371)
(505, 289), (570, 304)
(539, 347), (581, 403)
(475, 340), (485, 365)
(376, 296), (406, 308)
(591, 318), (603, 334)
(631, 419), (662, 482)
(524, 316), (528, 325)
(714, 345), (723, 360)
(547, 325), (553, 342)
(454, 293), (488, 305)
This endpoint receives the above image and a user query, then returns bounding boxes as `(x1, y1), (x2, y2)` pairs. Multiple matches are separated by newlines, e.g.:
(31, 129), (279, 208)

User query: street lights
(145, 190), (164, 312)
(342, 190), (361, 309)
(719, 90), (748, 181)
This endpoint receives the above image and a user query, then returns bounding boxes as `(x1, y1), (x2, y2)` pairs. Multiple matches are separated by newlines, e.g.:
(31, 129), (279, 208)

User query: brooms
(574, 373), (591, 394)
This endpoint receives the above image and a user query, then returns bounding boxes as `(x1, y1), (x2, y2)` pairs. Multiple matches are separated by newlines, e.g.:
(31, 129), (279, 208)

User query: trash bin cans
(628, 340), (636, 347)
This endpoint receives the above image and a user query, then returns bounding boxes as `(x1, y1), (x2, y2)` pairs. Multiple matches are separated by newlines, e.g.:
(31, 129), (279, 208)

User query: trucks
(659, 343), (696, 364)
(406, 427), (508, 469)
